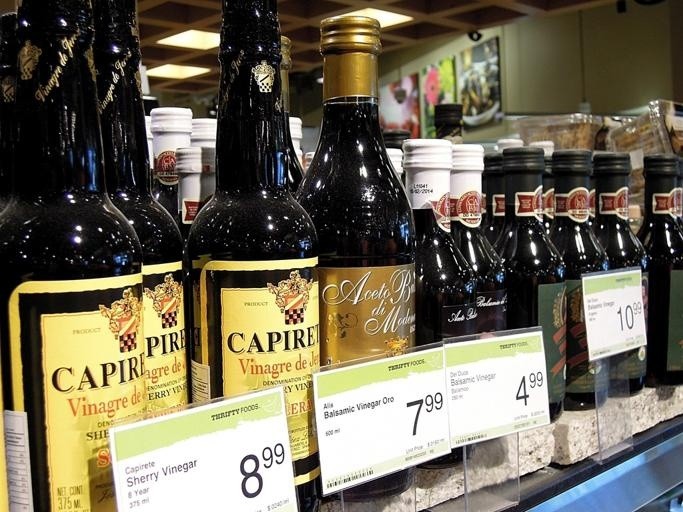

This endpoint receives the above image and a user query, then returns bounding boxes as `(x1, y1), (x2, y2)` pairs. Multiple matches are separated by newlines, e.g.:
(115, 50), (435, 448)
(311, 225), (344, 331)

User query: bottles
(0, 3), (682, 511)
